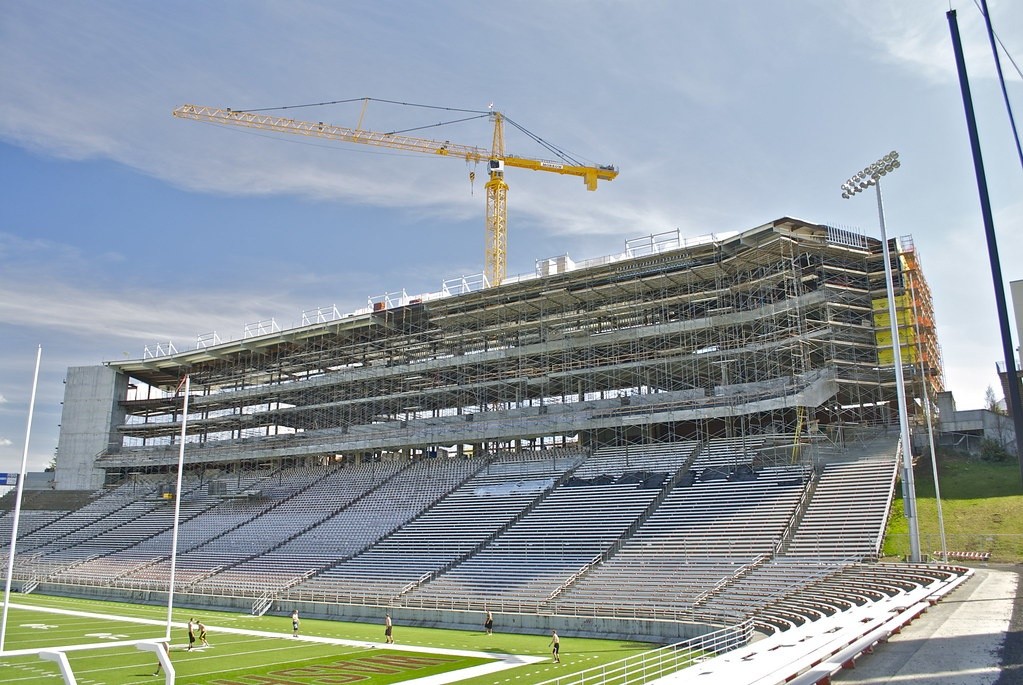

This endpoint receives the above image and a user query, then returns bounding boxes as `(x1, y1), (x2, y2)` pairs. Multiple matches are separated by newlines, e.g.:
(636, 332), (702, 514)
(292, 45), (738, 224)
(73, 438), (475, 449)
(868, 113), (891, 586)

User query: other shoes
(385, 640), (389, 643)
(392, 640), (394, 644)
(553, 660), (560, 664)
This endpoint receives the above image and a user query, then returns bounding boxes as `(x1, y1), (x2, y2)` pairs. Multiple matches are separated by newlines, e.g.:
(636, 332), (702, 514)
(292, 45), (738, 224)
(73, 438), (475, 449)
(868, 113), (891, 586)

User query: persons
(196, 621), (209, 648)
(484, 610), (493, 635)
(385, 613), (394, 644)
(292, 610), (300, 637)
(188, 618), (195, 651)
(153, 641), (169, 676)
(549, 630), (560, 663)
